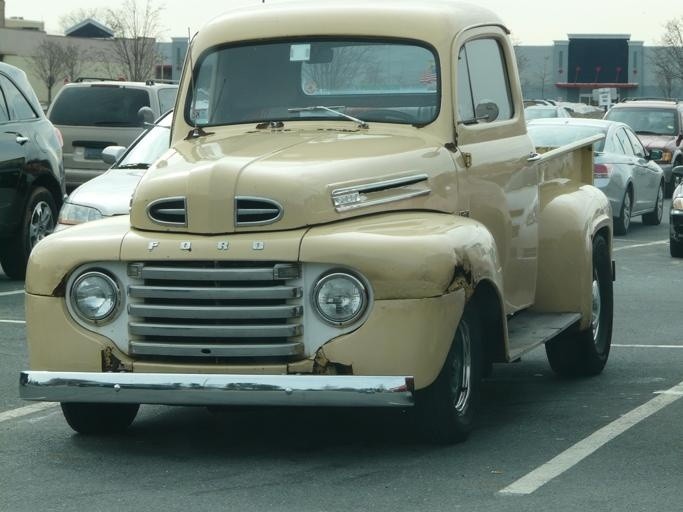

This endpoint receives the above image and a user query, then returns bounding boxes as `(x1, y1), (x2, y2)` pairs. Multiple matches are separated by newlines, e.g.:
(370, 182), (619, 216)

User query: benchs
(239, 106), (436, 123)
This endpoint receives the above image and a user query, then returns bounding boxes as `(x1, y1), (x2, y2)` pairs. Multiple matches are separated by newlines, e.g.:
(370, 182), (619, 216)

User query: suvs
(0, 59), (68, 277)
(45, 76), (212, 192)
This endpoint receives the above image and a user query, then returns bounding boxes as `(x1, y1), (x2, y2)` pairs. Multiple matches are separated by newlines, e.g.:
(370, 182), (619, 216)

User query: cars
(524, 96), (683, 260)
(18, 7), (617, 445)
